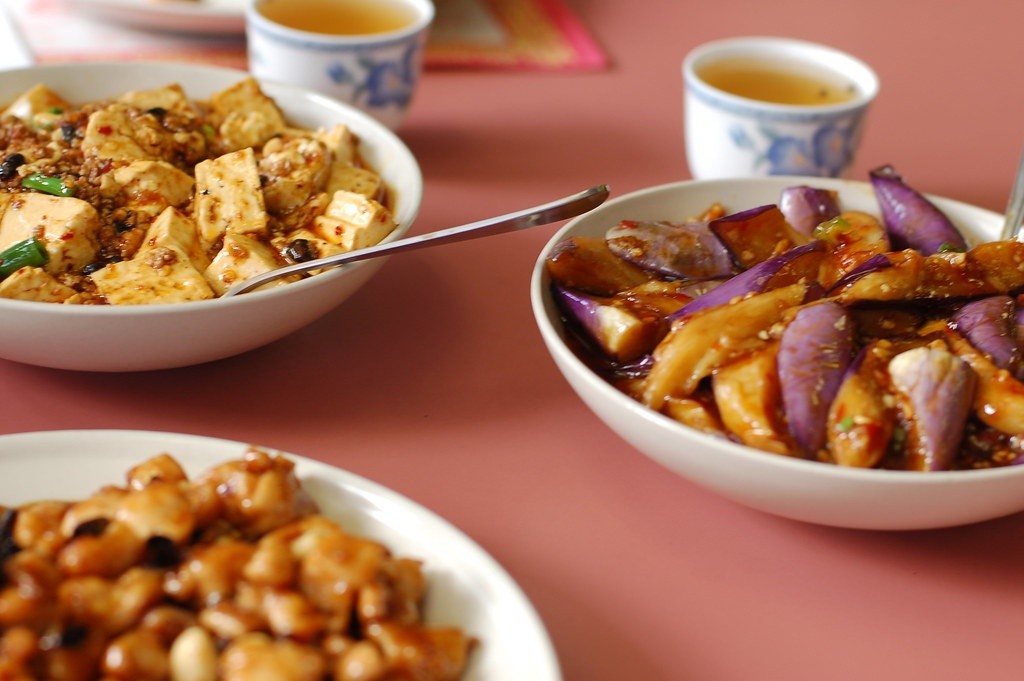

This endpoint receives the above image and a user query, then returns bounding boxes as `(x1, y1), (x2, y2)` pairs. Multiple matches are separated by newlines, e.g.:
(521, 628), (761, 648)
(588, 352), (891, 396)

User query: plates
(528, 174), (1024, 532)
(0, 62), (425, 371)
(64, 1), (247, 37)
(0, 428), (561, 681)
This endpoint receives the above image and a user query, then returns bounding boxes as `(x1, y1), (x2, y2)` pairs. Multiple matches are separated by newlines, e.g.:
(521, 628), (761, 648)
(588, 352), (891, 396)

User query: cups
(680, 36), (880, 179)
(245, 0), (436, 133)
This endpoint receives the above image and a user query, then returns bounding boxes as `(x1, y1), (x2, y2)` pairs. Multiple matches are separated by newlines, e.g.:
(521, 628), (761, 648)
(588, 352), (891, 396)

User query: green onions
(0, 173), (78, 281)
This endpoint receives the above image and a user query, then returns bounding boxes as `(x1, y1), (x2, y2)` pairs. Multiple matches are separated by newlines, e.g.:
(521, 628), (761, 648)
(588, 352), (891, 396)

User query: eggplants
(546, 164), (1024, 473)
